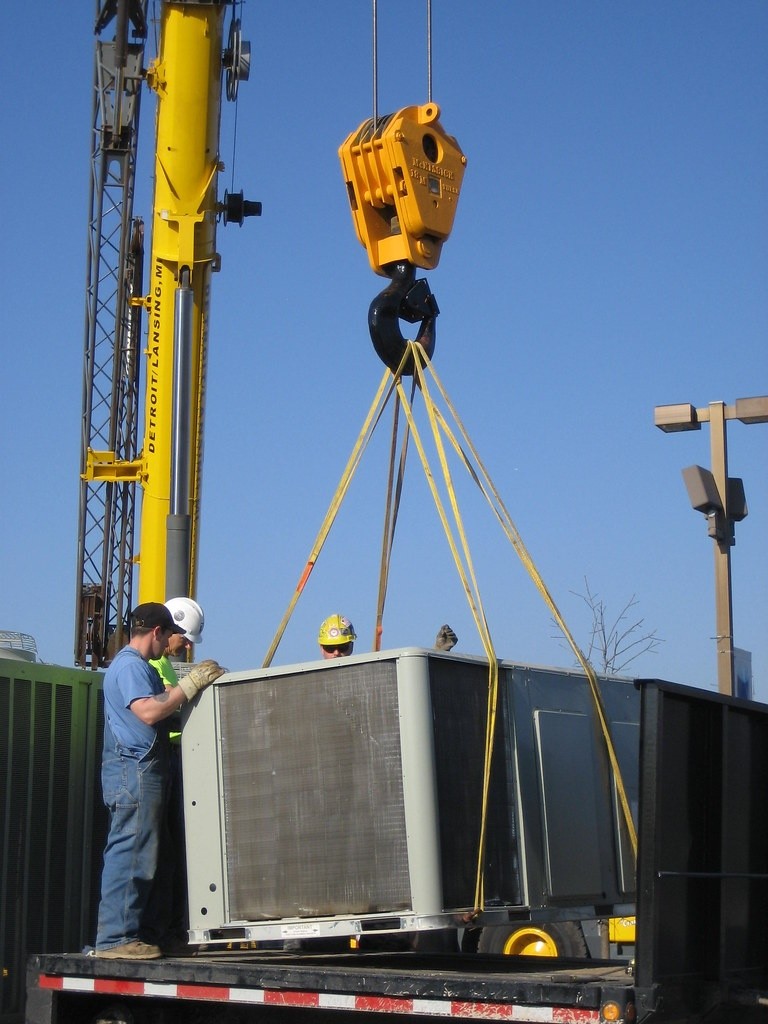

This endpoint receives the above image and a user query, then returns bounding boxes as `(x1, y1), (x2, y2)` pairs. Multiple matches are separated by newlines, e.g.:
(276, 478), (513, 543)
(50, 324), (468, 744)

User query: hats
(131, 603), (187, 634)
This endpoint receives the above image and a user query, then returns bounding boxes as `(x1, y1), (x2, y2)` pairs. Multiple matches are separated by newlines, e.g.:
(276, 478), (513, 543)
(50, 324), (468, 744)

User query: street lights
(649, 394), (768, 692)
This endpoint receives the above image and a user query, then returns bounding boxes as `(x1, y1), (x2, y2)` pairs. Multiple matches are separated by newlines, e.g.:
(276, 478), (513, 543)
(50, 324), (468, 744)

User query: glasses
(323, 642), (352, 653)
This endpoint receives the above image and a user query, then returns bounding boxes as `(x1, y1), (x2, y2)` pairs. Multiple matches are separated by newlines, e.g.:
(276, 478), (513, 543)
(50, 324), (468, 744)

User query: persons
(317, 613), (458, 659)
(95, 597), (226, 958)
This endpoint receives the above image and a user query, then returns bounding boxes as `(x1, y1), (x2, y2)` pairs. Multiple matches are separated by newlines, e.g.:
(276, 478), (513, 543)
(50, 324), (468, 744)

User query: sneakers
(153, 935), (195, 957)
(96, 938), (162, 959)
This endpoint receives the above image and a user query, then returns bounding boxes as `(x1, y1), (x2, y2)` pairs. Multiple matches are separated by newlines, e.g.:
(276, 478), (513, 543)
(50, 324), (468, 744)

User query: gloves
(434, 624), (457, 651)
(178, 660), (225, 702)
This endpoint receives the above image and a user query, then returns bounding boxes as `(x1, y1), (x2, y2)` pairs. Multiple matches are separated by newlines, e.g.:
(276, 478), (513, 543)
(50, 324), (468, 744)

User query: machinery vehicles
(74, 0), (467, 684)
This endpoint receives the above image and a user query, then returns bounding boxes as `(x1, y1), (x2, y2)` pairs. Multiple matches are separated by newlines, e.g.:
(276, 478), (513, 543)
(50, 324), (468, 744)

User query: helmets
(163, 596), (204, 643)
(318, 614), (356, 646)
